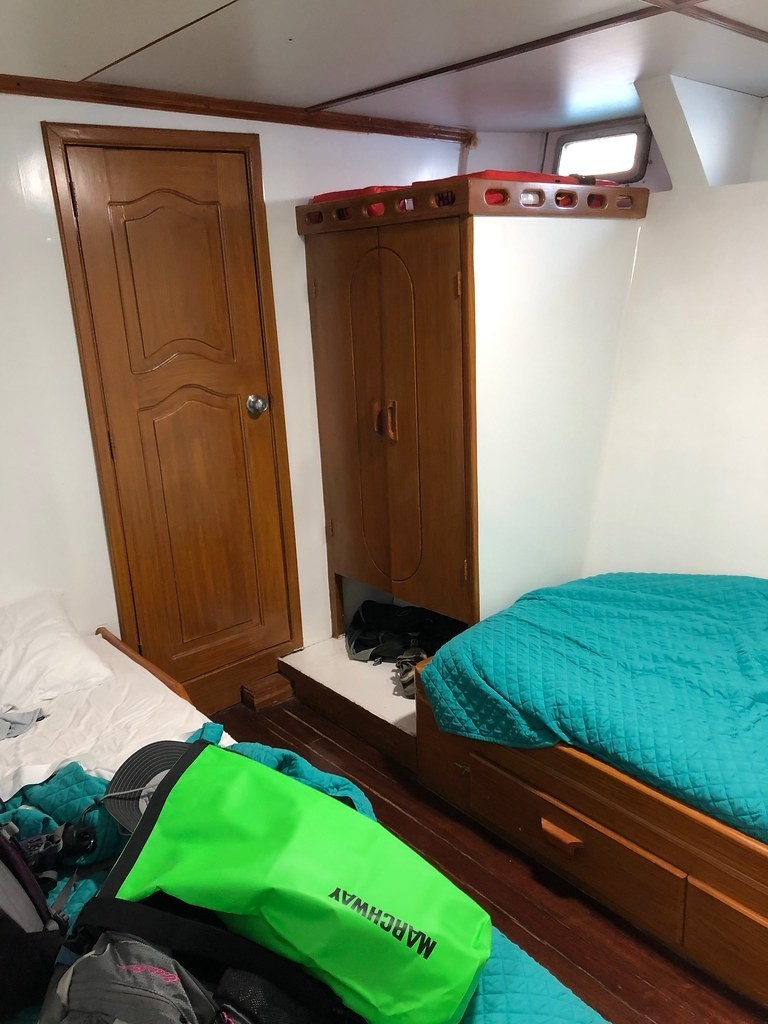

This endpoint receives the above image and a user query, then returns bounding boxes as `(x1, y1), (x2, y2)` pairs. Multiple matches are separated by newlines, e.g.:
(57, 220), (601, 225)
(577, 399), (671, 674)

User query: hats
(104, 741), (194, 833)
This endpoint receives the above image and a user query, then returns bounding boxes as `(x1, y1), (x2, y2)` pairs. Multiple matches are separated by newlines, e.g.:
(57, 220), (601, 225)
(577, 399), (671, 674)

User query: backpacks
(36, 931), (221, 1024)
(0, 821), (70, 1024)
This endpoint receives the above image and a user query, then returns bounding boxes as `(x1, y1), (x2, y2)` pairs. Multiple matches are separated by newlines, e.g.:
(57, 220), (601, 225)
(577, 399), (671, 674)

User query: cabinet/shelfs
(295, 179), (645, 639)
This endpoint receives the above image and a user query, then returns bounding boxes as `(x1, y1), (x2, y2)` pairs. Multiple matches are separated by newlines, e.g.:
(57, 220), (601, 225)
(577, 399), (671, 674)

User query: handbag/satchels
(345, 600), (468, 663)
(63, 739), (493, 1024)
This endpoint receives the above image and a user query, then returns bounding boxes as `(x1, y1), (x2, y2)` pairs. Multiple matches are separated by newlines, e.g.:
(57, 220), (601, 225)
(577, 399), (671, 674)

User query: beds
(0, 612), (610, 1024)
(409, 568), (768, 1009)
